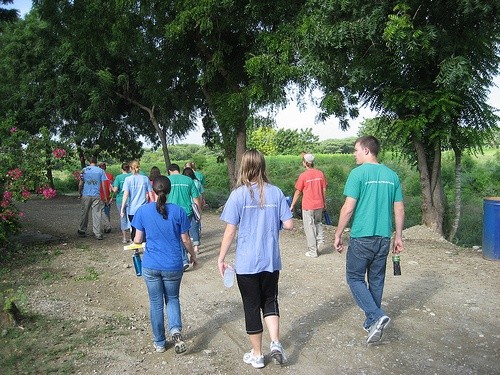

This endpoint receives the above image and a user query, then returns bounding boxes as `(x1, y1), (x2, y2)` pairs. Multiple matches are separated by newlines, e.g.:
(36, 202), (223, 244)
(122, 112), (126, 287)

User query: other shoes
(306, 250), (317, 258)
(318, 245), (324, 253)
(122, 239), (127, 243)
(194, 250), (200, 254)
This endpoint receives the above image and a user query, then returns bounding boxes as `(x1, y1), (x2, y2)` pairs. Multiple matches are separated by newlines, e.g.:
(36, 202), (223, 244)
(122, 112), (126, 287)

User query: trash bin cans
(482, 196), (500, 261)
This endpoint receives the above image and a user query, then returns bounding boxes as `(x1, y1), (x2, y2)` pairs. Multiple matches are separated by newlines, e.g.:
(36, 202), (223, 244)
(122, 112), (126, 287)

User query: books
(124, 242), (146, 251)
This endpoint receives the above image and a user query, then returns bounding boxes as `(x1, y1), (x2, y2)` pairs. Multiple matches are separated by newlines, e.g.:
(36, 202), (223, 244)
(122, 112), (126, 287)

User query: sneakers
(184, 263), (189, 270)
(366, 316), (391, 343)
(271, 341), (288, 366)
(363, 327), (371, 332)
(171, 329), (186, 354)
(106, 226), (112, 233)
(78, 230), (85, 234)
(154, 341), (168, 353)
(243, 349), (266, 369)
(94, 234), (104, 239)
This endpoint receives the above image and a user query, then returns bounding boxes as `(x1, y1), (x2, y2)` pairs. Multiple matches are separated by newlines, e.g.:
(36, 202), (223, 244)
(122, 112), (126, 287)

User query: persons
(109, 163), (147, 244)
(165, 164), (202, 272)
(145, 166), (162, 204)
(334, 136), (405, 343)
(98, 162), (113, 233)
(130, 176), (197, 354)
(78, 156), (108, 240)
(182, 161), (208, 254)
(120, 160), (155, 254)
(217, 150), (293, 369)
(290, 153), (328, 258)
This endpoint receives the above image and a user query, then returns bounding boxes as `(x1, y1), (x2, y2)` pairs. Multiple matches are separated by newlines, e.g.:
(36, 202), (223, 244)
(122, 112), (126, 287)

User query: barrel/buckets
(482, 197), (500, 261)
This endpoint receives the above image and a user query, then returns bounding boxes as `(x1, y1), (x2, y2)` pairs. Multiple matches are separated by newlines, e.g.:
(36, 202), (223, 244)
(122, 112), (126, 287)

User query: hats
(304, 154), (315, 164)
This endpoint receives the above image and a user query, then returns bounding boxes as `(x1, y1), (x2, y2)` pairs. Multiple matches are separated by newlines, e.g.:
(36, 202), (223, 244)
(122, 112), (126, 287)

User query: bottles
(323, 209), (330, 225)
(224, 268), (235, 288)
(392, 249), (401, 276)
(132, 249), (141, 276)
